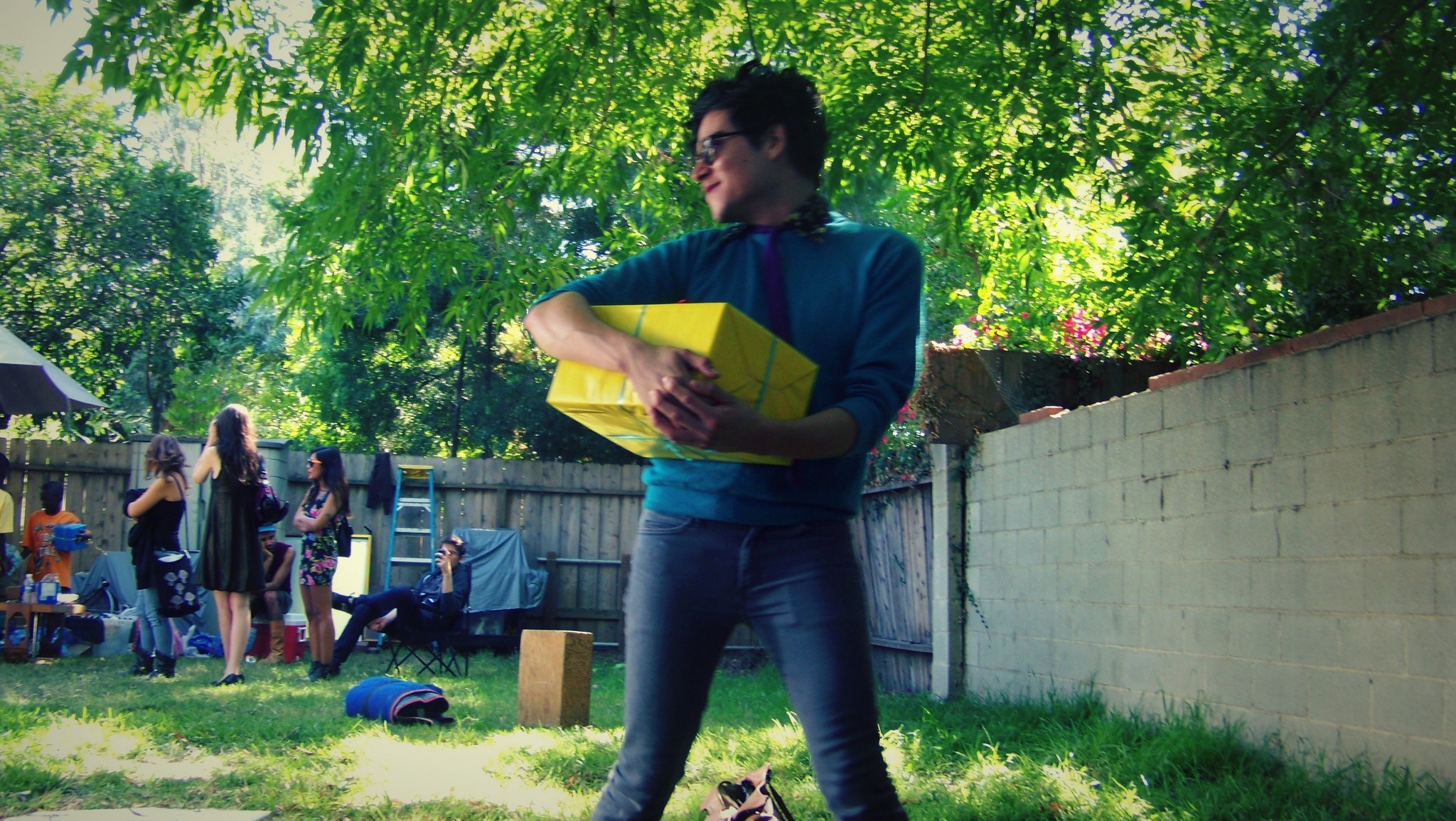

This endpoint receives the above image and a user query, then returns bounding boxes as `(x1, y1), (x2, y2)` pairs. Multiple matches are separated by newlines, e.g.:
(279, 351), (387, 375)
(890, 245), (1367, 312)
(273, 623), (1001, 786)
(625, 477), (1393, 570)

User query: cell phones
(438, 550), (445, 563)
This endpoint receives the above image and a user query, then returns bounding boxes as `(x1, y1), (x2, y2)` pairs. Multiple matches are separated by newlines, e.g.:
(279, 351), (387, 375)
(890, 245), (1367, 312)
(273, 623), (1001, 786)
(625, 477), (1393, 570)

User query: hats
(257, 525), (275, 532)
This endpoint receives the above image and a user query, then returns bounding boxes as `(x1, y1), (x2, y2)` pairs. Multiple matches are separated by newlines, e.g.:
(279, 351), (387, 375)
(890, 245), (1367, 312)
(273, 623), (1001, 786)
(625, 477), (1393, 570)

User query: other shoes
(296, 661), (335, 682)
(211, 673), (244, 685)
(330, 591), (353, 613)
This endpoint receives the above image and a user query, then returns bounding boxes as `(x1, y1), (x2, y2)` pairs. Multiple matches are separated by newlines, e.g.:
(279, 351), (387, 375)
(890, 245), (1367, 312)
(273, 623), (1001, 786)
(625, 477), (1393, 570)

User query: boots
(119, 646), (154, 676)
(145, 652), (177, 680)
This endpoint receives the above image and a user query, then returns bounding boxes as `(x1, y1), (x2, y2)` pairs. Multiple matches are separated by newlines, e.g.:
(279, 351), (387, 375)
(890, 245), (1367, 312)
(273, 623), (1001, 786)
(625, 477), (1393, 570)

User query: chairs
(387, 596), (470, 678)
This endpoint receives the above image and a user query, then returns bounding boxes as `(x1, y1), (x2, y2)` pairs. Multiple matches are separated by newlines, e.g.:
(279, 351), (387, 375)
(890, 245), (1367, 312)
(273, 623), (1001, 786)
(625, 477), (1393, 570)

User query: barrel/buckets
(244, 613), (308, 662)
(92, 615), (135, 658)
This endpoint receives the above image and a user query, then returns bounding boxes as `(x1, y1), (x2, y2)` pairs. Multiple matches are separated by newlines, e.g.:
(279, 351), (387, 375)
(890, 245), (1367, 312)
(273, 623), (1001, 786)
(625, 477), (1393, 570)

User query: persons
(192, 404), (264, 688)
(18, 479), (93, 662)
(329, 534), (472, 678)
(292, 446), (349, 684)
(0, 452), (16, 591)
(250, 522), (296, 666)
(122, 434), (187, 681)
(521, 58), (925, 821)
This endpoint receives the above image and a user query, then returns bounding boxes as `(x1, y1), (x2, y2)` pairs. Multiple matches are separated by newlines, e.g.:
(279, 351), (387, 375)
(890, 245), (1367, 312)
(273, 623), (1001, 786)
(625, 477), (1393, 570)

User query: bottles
(22, 574), (34, 604)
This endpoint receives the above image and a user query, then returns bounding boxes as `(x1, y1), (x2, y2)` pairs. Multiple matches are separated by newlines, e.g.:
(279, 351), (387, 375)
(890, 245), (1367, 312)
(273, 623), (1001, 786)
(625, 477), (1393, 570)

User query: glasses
(693, 126), (768, 165)
(308, 458), (322, 468)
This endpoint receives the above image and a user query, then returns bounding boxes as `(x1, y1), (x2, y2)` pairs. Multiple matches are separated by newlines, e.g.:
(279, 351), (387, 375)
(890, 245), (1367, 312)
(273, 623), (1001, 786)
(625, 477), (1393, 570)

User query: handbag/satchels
(256, 457), (290, 526)
(698, 763), (789, 821)
(153, 553), (200, 619)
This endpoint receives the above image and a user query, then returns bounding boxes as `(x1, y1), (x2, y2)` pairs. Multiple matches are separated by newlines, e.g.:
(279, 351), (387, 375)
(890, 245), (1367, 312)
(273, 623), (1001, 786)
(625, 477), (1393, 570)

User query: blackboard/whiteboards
(284, 534), (372, 641)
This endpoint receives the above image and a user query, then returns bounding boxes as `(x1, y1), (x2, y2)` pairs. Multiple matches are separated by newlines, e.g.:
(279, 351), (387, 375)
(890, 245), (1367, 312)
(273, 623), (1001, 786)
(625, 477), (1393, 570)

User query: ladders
(376, 464), (437, 651)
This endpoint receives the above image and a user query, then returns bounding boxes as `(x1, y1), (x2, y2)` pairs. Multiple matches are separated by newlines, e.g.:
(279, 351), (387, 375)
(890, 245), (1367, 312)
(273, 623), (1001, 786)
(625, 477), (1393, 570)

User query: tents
(0, 324), (111, 411)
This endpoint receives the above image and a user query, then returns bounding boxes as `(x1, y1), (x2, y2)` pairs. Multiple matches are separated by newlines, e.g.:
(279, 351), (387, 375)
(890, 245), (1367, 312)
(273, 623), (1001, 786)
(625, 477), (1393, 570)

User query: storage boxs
(244, 612), (307, 663)
(546, 302), (818, 465)
(519, 629), (593, 728)
(89, 616), (133, 659)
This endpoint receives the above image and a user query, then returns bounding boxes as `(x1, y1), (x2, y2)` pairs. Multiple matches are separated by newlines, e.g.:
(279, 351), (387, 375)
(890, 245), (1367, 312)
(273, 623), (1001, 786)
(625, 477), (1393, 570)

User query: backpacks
(321, 489), (351, 558)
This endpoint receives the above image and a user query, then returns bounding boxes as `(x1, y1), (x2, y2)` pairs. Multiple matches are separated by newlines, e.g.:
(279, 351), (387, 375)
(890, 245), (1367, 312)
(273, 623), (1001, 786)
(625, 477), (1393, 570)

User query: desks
(0, 603), (85, 663)
